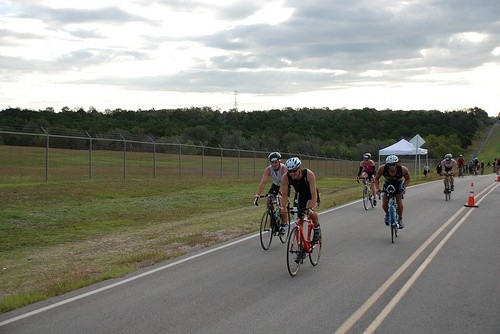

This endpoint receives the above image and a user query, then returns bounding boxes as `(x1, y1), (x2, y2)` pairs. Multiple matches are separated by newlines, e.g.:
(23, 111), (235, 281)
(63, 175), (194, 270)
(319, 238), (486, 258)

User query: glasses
(288, 167), (300, 173)
(271, 161), (278, 164)
(389, 163), (397, 166)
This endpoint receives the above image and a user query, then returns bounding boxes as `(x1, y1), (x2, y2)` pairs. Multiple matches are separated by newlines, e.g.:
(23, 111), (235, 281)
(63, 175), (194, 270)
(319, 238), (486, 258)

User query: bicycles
(376, 185), (406, 243)
(440, 170), (455, 201)
(356, 173), (378, 210)
(278, 203), (322, 278)
(458, 163), (485, 176)
(253, 190), (291, 250)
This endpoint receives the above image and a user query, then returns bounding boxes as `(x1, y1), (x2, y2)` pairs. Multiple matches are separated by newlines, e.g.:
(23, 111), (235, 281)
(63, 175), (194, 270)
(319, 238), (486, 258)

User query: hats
(270, 157), (282, 161)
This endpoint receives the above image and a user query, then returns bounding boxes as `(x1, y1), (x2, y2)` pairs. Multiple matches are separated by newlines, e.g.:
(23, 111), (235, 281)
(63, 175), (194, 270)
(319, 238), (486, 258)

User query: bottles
(273, 210), (278, 224)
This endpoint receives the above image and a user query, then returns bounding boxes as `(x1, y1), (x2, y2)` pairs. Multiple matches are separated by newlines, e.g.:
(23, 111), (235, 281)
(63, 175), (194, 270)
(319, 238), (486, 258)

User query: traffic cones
(494, 175), (500, 182)
(463, 182), (478, 207)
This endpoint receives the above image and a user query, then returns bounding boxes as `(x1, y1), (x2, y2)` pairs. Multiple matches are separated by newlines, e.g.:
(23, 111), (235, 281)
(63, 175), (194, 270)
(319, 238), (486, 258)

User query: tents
(379, 139), (428, 179)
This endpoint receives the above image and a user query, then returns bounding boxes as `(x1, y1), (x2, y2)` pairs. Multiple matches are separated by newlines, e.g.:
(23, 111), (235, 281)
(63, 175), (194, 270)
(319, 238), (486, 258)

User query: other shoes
(398, 217), (404, 228)
(373, 197), (377, 206)
(279, 223), (288, 234)
(385, 211), (389, 226)
(451, 184), (454, 191)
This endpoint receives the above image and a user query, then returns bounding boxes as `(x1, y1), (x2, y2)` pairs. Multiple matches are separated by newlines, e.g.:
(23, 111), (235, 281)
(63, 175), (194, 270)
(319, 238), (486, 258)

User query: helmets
(285, 157), (302, 170)
(386, 155), (399, 164)
(364, 153), (371, 158)
(444, 154), (453, 158)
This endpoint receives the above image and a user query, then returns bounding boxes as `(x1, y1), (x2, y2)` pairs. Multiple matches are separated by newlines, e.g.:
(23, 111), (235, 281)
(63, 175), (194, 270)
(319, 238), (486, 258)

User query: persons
(473, 157), (484, 175)
(486, 157), (500, 172)
(280, 157), (321, 265)
(254, 152), (291, 235)
(423, 164), (431, 179)
(457, 155), (465, 175)
(358, 152), (377, 206)
(440, 154), (457, 194)
(375, 156), (410, 228)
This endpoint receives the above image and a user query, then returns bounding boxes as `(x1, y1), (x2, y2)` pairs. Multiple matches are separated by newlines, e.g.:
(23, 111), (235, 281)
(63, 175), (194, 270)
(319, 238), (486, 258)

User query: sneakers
(312, 222), (320, 241)
(295, 252), (307, 263)
